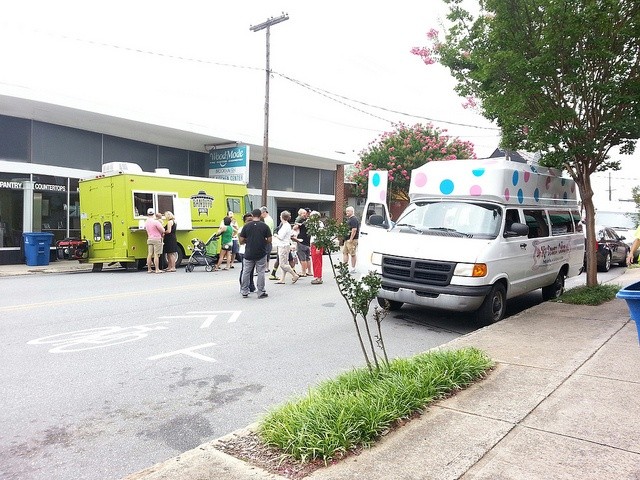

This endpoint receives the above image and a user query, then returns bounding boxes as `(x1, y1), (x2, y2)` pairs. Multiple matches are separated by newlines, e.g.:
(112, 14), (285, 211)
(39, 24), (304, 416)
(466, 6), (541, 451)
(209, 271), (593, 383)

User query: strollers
(185, 233), (219, 272)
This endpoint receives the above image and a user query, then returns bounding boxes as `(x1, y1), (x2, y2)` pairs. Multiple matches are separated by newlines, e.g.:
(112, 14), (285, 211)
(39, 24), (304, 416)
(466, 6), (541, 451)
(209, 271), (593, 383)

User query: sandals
(306, 273), (313, 276)
(292, 276), (300, 284)
(298, 273), (307, 277)
(275, 281), (285, 284)
(311, 279), (323, 284)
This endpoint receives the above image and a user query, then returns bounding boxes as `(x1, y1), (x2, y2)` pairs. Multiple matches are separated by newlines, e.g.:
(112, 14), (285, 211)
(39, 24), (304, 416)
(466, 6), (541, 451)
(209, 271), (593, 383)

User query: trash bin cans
(23, 231), (54, 266)
(617, 282), (640, 343)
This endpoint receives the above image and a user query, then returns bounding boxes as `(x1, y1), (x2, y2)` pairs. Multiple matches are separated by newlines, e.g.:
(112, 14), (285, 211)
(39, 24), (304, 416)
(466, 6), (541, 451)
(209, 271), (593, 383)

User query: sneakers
(258, 293), (268, 298)
(295, 273), (301, 277)
(336, 267), (344, 272)
(218, 265), (221, 269)
(349, 268), (356, 274)
(242, 295), (248, 298)
(230, 264), (235, 268)
(269, 276), (280, 280)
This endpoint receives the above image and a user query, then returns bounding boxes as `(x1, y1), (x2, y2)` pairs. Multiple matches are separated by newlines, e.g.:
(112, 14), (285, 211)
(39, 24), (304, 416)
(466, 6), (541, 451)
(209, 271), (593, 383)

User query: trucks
(78, 162), (253, 272)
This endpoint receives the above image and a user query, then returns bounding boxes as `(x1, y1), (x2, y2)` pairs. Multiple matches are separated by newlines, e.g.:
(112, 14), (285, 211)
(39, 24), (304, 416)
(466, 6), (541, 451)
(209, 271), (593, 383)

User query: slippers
(155, 270), (165, 273)
(212, 268), (218, 271)
(163, 268), (169, 271)
(148, 270), (155, 273)
(222, 267), (229, 270)
(166, 269), (176, 272)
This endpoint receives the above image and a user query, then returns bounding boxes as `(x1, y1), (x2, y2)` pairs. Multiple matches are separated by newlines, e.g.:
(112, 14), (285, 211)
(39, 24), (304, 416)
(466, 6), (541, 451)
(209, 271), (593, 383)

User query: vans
(356, 160), (584, 327)
(581, 203), (639, 263)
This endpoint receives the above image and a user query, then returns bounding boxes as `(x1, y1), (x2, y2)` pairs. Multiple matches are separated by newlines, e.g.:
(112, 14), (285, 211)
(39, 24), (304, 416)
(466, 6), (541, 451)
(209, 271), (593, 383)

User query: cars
(584, 226), (630, 271)
(237, 221), (339, 261)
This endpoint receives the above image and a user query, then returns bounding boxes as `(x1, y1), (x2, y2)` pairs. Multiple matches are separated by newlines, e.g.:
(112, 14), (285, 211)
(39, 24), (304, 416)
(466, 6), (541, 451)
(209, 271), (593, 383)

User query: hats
(147, 208), (154, 214)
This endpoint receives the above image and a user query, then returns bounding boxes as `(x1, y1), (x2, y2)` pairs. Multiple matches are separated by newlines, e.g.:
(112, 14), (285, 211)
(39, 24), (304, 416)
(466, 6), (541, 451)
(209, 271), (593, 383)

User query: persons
(163, 210), (177, 272)
(269, 210), (304, 281)
(382, 213), (396, 224)
(293, 206), (312, 277)
(625, 222), (640, 273)
(294, 208), (305, 226)
(274, 210), (300, 286)
(309, 210), (325, 286)
(238, 214), (257, 293)
(220, 210), (238, 271)
(144, 206), (165, 275)
(339, 202), (358, 276)
(259, 206), (274, 272)
(155, 212), (164, 227)
(214, 216), (238, 271)
(239, 209), (273, 299)
(231, 217), (238, 231)
